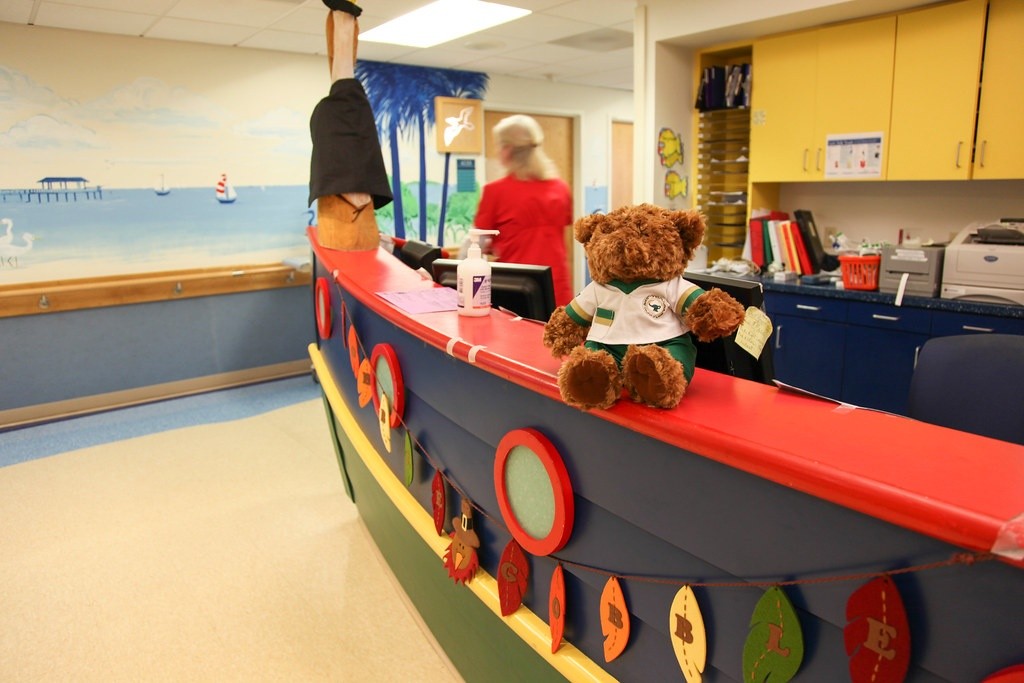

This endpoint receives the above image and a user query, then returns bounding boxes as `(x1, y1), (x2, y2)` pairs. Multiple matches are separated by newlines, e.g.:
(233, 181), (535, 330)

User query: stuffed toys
(543, 203), (746, 410)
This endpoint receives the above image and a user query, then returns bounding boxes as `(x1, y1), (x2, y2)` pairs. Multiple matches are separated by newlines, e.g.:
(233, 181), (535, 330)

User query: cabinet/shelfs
(842, 298), (934, 418)
(931, 310), (1023, 340)
(692, 37), (779, 265)
(764, 290), (844, 404)
(886, 0), (1024, 180)
(747, 14), (896, 184)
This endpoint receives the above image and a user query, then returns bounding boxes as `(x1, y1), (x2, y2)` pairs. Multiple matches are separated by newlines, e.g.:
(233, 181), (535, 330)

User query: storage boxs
(838, 255), (880, 289)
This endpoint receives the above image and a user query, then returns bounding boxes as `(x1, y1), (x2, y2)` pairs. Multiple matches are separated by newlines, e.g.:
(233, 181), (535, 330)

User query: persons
(473, 115), (574, 307)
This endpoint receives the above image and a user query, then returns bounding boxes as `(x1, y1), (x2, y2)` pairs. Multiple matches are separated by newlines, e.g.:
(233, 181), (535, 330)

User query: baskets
(838, 256), (880, 290)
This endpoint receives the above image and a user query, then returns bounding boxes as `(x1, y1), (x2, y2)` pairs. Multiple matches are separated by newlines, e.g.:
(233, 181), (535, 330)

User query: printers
(939, 216), (1024, 306)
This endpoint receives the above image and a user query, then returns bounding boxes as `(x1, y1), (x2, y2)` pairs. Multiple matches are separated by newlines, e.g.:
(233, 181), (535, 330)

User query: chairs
(909, 332), (1024, 448)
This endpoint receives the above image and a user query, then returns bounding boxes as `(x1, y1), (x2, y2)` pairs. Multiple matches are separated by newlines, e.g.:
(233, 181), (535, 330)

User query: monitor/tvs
(433, 258), (557, 323)
(681, 272), (776, 385)
(402, 241), (441, 279)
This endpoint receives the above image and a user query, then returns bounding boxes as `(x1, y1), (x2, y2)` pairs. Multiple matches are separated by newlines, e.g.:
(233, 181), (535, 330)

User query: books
(749, 209), (825, 275)
